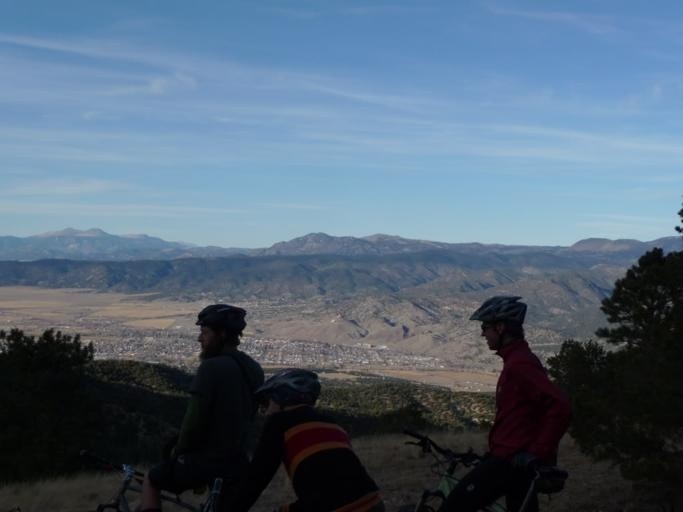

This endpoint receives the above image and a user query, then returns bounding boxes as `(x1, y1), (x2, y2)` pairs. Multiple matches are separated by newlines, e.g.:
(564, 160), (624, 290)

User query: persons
(129, 302), (266, 512)
(208, 365), (387, 511)
(433, 293), (574, 511)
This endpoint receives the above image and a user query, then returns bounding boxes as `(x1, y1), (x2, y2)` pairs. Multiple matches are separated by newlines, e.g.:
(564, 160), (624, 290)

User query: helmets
(254, 368), (321, 406)
(469, 295), (527, 324)
(195, 304), (247, 328)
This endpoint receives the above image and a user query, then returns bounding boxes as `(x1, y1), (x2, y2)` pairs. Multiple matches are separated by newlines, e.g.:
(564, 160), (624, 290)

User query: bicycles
(399, 428), (569, 512)
(201, 476), (225, 512)
(76, 446), (204, 512)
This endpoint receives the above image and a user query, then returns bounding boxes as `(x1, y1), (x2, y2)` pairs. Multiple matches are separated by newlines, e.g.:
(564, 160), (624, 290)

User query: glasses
(481, 325), (494, 332)
